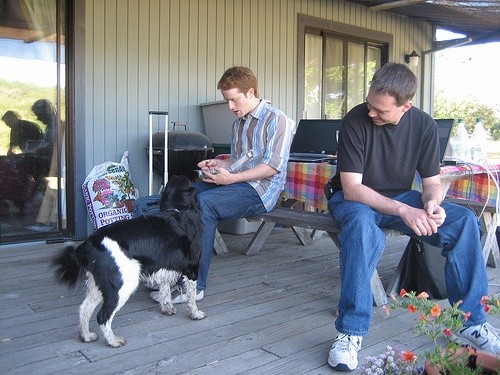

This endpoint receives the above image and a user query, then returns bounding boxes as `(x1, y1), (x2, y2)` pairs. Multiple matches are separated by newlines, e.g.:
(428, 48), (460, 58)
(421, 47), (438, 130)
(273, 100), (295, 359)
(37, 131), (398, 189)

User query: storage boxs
(198, 99), (273, 159)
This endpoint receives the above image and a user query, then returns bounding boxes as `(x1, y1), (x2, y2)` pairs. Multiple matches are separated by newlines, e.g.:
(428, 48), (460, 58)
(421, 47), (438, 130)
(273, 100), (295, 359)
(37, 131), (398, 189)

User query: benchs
(445, 195), (497, 214)
(244, 204), (402, 239)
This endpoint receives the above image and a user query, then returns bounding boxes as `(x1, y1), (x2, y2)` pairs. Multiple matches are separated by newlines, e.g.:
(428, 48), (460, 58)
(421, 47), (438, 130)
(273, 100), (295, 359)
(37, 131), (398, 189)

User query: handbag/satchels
(412, 237), (451, 301)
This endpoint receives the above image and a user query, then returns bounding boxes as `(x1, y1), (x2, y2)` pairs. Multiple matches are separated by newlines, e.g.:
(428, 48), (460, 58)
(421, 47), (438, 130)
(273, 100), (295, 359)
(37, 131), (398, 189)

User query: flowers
(381, 287), (500, 375)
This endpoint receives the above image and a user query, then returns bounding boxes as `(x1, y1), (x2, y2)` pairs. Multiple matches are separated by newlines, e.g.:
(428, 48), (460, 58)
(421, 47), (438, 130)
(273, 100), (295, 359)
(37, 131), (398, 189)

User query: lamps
(405, 50), (421, 68)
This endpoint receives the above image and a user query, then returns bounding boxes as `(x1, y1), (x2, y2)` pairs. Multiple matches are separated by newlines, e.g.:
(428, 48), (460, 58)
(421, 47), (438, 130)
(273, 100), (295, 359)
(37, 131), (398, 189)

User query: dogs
(48, 174), (208, 348)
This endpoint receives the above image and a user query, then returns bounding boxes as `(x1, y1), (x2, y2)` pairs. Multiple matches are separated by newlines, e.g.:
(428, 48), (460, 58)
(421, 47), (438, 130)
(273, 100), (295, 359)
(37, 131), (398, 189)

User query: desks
(241, 163), (500, 306)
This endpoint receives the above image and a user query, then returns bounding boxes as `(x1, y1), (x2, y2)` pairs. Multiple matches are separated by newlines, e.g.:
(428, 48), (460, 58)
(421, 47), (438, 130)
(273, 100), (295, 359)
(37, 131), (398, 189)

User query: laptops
(289, 119), (342, 162)
(434, 119), (454, 167)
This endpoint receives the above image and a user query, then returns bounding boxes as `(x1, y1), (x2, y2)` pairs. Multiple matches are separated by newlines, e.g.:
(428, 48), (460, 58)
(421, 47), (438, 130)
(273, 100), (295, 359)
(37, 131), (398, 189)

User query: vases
(425, 347), (500, 374)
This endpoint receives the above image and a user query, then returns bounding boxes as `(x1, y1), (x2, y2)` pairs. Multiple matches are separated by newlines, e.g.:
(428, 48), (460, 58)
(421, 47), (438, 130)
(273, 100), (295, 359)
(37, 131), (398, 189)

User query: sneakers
(328, 332), (364, 372)
(447, 323), (500, 357)
(144, 280), (206, 304)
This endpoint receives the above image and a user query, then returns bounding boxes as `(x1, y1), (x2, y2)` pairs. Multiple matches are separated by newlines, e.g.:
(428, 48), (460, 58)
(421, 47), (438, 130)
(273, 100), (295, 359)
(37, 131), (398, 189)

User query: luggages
(134, 110), (170, 220)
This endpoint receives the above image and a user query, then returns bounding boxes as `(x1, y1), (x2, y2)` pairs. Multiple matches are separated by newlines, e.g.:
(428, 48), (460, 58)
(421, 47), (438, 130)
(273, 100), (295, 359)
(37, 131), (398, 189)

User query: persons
(146, 66), (293, 304)
(1, 110), (44, 157)
(26, 99), (67, 205)
(327, 62), (499, 371)
